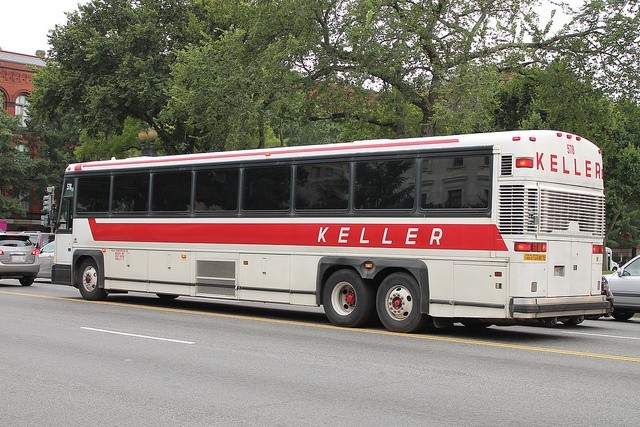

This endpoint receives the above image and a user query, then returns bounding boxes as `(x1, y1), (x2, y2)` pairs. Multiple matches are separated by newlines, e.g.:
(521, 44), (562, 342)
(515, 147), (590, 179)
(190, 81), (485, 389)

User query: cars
(0, 231), (54, 286)
(211, 256), (603, 305)
(602, 253), (640, 322)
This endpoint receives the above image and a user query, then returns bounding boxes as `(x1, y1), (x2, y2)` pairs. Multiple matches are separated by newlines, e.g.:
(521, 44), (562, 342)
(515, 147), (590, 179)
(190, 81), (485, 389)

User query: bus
(52, 128), (611, 334)
